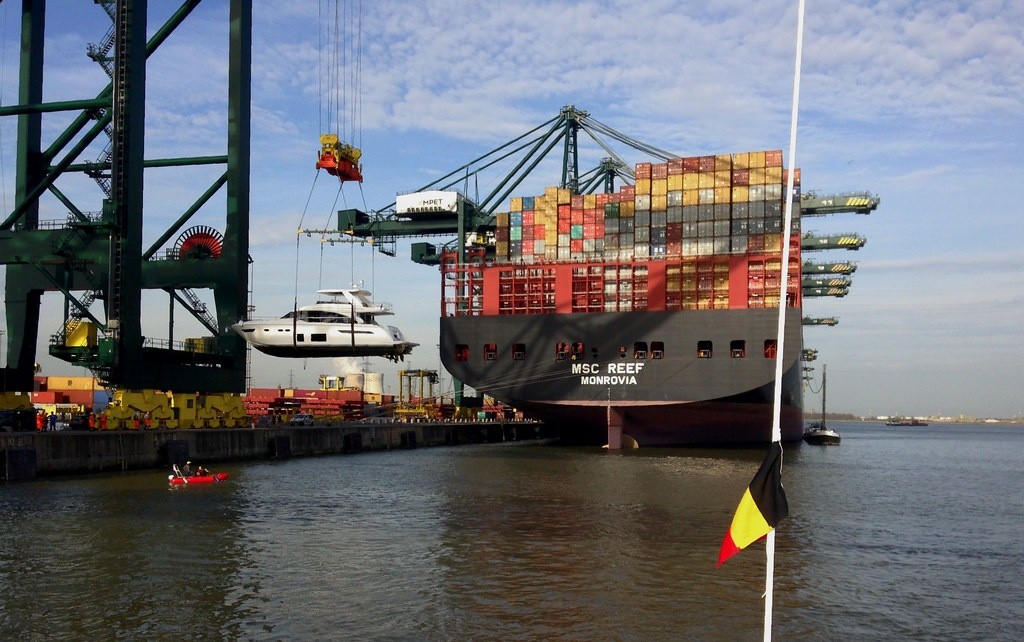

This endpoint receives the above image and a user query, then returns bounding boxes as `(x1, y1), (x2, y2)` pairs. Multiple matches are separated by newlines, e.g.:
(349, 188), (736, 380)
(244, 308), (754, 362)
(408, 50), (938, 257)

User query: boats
(166, 463), (228, 487)
(885, 417), (929, 427)
(228, 287), (419, 366)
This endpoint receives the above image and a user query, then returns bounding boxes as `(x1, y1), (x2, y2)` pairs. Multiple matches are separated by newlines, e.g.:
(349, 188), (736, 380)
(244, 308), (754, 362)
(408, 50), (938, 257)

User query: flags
(716, 440), (789, 571)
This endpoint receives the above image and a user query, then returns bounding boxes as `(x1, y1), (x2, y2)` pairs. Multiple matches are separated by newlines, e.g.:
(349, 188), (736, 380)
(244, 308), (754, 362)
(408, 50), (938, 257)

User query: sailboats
(805, 363), (842, 447)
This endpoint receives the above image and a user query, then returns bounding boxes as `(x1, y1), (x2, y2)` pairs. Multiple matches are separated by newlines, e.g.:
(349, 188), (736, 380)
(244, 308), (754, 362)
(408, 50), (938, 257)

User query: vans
(290, 414), (315, 426)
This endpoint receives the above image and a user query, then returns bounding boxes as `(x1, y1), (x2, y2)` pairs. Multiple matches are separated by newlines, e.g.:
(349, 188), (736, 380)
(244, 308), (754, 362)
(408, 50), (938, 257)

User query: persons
(183, 461), (192, 476)
(195, 466), (209, 476)
(36, 408), (282, 431)
